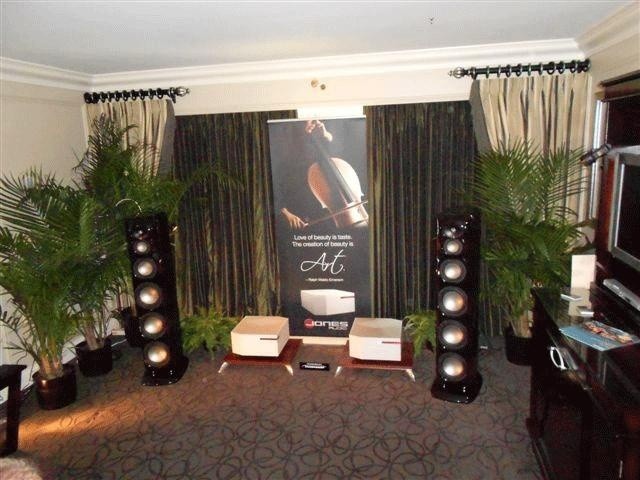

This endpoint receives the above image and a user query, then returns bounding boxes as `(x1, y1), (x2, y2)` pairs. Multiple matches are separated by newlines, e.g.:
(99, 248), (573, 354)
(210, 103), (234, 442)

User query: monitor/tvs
(605, 147), (640, 297)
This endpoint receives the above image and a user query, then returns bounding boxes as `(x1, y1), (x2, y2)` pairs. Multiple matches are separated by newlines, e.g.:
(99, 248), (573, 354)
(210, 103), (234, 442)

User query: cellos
(304, 120), (370, 230)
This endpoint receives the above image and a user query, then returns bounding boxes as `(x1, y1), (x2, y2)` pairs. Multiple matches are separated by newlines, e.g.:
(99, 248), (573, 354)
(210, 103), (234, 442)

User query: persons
(268, 120), (332, 337)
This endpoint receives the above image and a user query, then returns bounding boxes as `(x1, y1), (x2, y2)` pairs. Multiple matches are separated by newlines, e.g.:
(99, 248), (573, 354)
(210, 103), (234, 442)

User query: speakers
(430, 207), (484, 406)
(123, 211), (190, 386)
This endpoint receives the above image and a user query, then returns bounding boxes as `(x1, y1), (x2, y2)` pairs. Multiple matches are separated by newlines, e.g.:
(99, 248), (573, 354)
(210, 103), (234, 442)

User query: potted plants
(0, 196), (79, 411)
(464, 133), (598, 365)
(73, 113), (255, 350)
(23, 164), (128, 378)
(179, 303), (239, 351)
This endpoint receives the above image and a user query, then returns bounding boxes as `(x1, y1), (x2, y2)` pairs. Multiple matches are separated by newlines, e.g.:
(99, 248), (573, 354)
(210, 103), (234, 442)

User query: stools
(0, 363), (25, 458)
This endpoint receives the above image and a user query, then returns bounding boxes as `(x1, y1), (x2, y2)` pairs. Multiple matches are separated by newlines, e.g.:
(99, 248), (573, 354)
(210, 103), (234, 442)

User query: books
(558, 321), (640, 352)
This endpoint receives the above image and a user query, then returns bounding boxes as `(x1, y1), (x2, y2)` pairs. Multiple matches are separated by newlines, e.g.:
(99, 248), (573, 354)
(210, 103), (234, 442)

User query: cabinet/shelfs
(526, 149), (640, 480)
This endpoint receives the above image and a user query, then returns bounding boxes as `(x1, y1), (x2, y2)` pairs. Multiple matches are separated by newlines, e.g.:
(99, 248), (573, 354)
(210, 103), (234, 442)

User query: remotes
(577, 306), (594, 315)
(561, 293), (583, 302)
